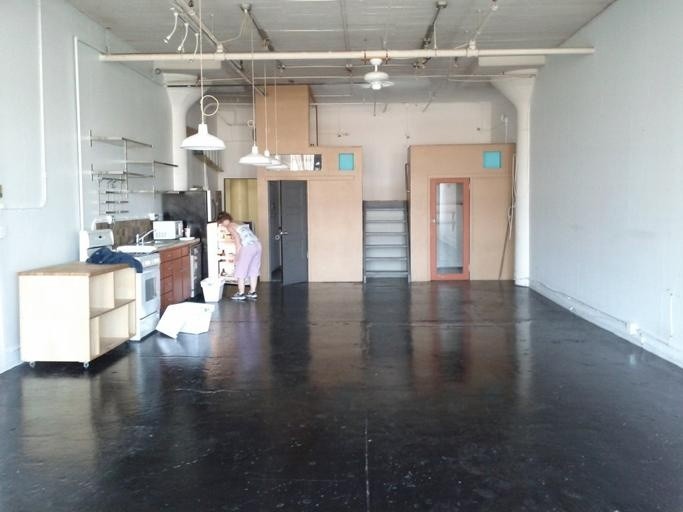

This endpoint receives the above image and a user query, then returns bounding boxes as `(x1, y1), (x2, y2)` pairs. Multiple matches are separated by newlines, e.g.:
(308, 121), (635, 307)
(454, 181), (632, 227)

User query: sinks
(140, 240), (171, 246)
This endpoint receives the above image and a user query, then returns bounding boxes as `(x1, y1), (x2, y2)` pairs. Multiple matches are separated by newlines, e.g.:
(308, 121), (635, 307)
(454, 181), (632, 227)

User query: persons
(217, 212), (262, 300)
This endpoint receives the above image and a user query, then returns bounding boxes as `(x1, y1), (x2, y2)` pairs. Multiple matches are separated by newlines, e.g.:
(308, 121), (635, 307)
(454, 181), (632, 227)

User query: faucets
(136, 229), (157, 242)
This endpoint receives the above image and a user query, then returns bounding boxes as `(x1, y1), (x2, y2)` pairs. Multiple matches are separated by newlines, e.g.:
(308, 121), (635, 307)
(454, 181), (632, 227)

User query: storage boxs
(171, 301), (215, 334)
(199, 277), (226, 302)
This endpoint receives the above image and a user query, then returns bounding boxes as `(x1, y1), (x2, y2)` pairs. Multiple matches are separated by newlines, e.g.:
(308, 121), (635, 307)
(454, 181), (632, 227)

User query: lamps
(236, 3), (287, 171)
(180, 1), (225, 152)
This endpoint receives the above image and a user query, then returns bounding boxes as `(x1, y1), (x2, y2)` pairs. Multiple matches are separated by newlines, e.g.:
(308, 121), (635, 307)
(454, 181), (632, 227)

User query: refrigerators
(163, 189), (252, 286)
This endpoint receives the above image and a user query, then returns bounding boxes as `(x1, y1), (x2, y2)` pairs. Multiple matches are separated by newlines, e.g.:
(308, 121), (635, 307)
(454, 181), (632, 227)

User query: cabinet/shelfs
(88, 129), (179, 216)
(18, 262), (135, 369)
(160, 246), (190, 312)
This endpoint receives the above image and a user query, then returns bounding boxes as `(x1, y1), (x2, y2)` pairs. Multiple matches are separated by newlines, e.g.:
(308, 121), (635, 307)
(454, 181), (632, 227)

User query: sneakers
(231, 292), (247, 299)
(246, 292), (258, 298)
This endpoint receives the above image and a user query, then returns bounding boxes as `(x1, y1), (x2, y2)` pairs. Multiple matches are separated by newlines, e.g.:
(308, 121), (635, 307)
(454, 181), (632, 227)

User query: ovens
(133, 255), (162, 344)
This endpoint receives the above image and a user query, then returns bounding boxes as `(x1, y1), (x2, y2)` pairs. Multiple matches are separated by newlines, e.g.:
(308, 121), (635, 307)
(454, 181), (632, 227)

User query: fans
(318, 58), (430, 91)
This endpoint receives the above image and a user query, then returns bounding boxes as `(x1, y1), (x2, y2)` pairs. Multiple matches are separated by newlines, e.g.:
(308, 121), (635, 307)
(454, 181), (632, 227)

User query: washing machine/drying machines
(78, 228), (161, 343)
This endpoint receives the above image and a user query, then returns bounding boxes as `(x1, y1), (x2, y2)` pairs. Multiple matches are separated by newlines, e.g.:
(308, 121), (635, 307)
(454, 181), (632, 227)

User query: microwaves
(151, 220), (184, 239)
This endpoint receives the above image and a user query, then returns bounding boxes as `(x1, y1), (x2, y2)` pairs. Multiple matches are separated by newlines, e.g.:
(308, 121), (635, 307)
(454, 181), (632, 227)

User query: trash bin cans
(200, 277), (224, 303)
